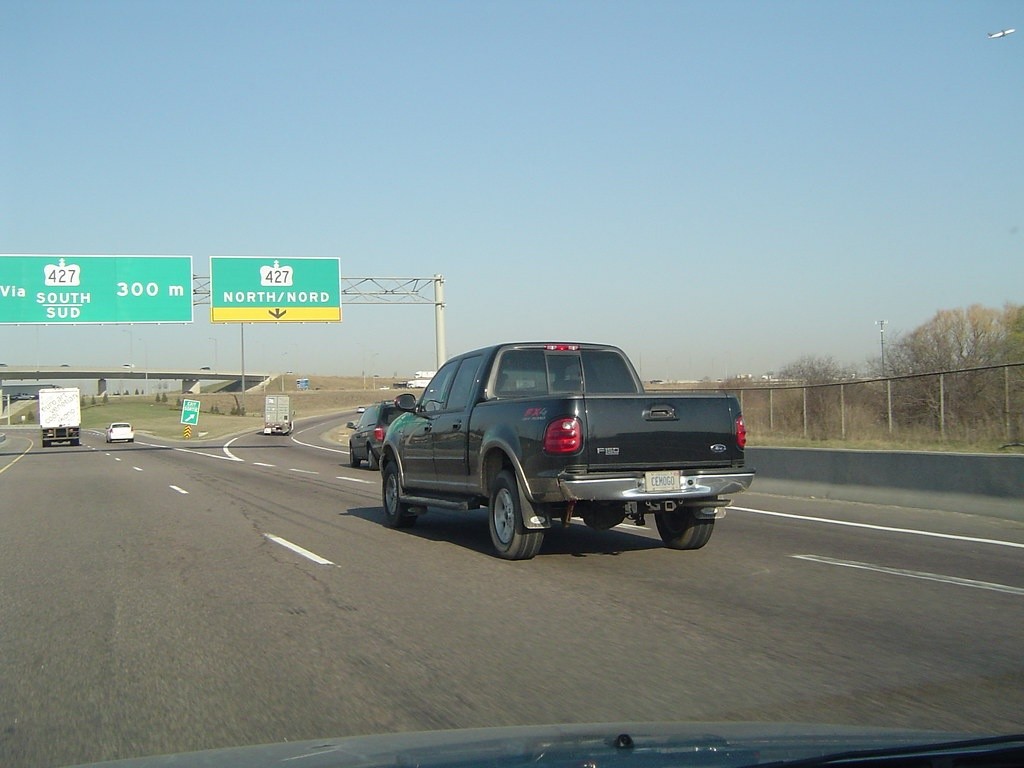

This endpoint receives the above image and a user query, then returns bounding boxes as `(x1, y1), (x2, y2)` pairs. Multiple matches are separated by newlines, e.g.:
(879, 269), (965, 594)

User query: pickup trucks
(378, 340), (757, 561)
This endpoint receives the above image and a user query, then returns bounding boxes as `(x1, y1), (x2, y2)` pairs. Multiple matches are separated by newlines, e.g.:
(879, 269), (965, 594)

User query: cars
(357, 406), (366, 413)
(105, 422), (135, 443)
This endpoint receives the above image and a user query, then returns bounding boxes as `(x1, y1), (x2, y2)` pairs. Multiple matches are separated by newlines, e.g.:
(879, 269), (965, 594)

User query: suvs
(347, 399), (427, 471)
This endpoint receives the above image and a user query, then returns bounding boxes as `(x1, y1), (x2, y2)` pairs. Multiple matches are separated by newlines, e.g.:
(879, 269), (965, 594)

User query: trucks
(262, 394), (293, 436)
(39, 387), (81, 447)
